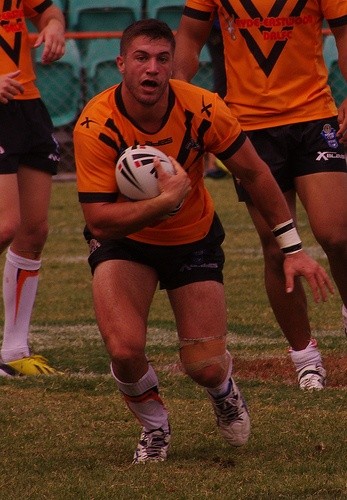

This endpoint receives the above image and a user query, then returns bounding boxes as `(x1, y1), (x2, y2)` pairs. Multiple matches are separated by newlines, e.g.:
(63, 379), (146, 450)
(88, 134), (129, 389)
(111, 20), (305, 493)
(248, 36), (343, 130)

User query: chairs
(25, 0), (347, 126)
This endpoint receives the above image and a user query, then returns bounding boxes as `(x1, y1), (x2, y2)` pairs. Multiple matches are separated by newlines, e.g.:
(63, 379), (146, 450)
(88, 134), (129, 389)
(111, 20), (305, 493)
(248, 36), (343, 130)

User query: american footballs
(115, 143), (184, 219)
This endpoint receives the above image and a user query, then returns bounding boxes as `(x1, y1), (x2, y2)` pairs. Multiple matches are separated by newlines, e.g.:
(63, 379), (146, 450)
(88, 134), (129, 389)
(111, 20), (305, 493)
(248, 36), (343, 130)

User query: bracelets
(271, 219), (304, 255)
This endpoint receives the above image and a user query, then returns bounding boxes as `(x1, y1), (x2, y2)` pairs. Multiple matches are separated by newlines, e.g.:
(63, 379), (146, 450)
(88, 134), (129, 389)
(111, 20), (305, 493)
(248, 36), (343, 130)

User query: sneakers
(297, 365), (326, 393)
(131, 420), (172, 464)
(0, 355), (72, 382)
(207, 377), (250, 447)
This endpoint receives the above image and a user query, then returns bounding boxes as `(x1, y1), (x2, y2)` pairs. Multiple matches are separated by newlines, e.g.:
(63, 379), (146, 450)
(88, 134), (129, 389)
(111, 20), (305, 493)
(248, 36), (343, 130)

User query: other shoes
(204, 167), (227, 178)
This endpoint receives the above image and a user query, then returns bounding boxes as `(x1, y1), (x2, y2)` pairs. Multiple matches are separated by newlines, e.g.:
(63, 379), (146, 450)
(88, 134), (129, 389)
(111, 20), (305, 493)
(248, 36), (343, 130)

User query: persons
(74, 17), (335, 464)
(171, 0), (347, 391)
(0, 0), (66, 378)
